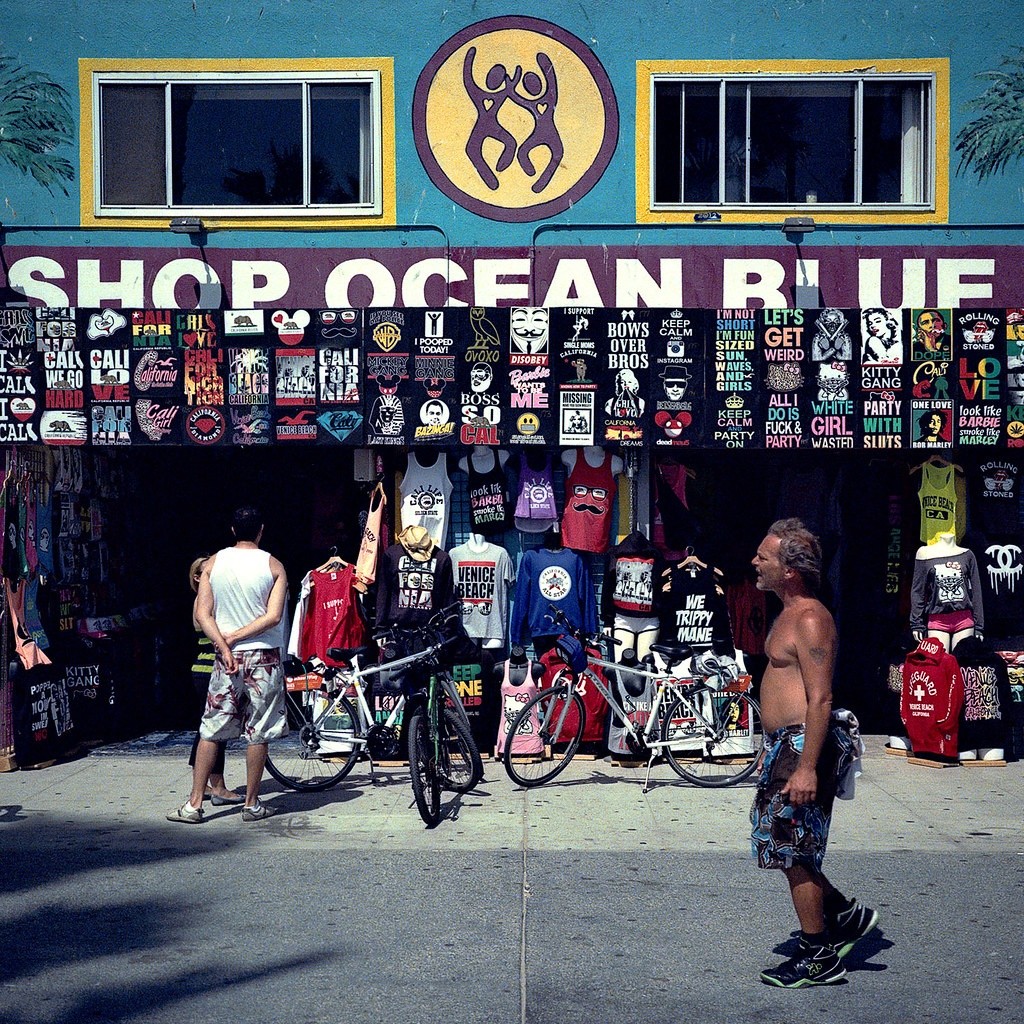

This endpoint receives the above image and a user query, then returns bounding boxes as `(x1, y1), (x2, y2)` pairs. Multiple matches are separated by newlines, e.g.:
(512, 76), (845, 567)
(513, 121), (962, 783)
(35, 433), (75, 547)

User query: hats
(398, 524), (437, 563)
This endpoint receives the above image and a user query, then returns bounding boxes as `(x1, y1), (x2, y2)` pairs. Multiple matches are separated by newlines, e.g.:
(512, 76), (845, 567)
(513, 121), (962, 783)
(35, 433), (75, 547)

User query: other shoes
(211, 793), (248, 805)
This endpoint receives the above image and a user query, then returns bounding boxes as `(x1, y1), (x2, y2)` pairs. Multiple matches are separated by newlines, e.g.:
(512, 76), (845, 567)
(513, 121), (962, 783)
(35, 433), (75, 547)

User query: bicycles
(503, 606), (769, 793)
(370, 596), (483, 830)
(263, 614), (476, 794)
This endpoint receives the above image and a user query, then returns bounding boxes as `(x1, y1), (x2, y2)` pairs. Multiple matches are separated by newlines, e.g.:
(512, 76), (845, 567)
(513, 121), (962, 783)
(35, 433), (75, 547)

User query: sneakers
(241, 803), (280, 821)
(830, 897), (879, 957)
(166, 804), (204, 824)
(759, 939), (848, 989)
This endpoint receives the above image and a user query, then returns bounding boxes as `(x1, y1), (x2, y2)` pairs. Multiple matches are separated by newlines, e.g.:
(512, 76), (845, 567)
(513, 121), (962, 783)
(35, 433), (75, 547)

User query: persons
(890, 530), (1007, 762)
(188, 557), (245, 805)
(167, 506), (289, 823)
(487, 646), (546, 758)
(600, 529), (662, 665)
(745, 518), (879, 986)
(372, 522), (453, 653)
(602, 647), (658, 762)
(448, 534), (517, 649)
(426, 401), (443, 426)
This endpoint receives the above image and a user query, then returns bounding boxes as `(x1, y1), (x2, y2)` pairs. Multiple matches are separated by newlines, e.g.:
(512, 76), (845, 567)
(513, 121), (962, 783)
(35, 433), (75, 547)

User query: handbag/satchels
(555, 634), (588, 672)
